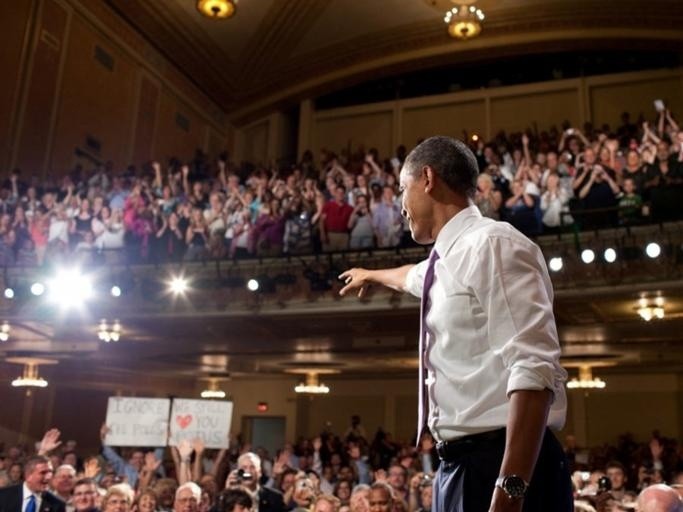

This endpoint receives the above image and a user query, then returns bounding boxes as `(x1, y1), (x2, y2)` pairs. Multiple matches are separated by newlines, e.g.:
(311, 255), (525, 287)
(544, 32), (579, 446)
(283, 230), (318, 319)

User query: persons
(0, 412), (683, 511)
(1, 99), (681, 271)
(337, 135), (573, 511)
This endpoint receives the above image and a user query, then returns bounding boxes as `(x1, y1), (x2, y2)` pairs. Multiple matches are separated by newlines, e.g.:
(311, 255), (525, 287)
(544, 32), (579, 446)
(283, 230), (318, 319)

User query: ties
(25, 497), (36, 512)
(416, 249), (439, 449)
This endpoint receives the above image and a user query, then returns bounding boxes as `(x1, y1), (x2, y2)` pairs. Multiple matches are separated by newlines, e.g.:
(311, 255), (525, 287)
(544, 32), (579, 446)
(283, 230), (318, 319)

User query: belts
(436, 432), (501, 460)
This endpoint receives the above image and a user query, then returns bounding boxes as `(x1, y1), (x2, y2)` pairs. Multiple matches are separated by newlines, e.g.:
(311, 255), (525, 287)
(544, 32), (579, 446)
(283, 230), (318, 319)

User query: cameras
(233, 469), (253, 481)
(419, 473), (433, 486)
(597, 476), (612, 493)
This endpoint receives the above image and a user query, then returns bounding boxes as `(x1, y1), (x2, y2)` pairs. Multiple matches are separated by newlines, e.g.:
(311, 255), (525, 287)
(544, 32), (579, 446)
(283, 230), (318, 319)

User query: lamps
(6, 359), (60, 392)
(195, 0), (238, 21)
(197, 359), (232, 399)
(281, 359), (340, 395)
(441, 0), (487, 42)
